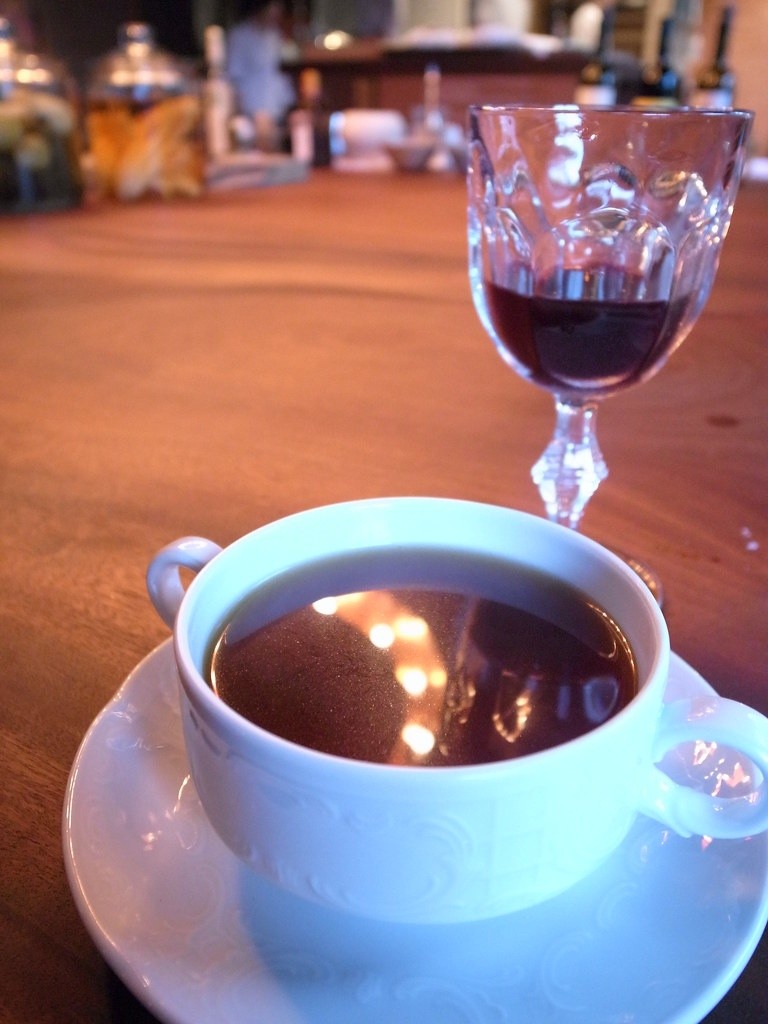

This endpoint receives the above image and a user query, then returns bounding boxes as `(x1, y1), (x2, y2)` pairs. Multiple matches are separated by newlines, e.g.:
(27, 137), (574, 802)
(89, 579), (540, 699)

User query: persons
(230, 6), (294, 123)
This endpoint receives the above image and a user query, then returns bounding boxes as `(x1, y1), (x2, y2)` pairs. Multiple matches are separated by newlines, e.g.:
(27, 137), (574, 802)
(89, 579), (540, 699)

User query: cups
(146, 496), (768, 924)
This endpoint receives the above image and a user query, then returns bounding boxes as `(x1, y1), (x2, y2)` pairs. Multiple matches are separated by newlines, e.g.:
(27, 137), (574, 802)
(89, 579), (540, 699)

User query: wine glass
(468, 104), (756, 607)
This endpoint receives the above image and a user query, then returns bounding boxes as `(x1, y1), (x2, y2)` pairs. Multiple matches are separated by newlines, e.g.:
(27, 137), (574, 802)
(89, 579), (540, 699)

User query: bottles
(0, 16), (198, 216)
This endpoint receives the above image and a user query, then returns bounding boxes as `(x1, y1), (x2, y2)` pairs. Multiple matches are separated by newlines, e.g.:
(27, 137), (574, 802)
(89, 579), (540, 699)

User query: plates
(62, 637), (768, 1024)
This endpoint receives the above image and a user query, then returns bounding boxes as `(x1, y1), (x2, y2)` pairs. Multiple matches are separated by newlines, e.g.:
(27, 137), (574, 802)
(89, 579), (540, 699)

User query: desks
(0, 171), (768, 1024)
